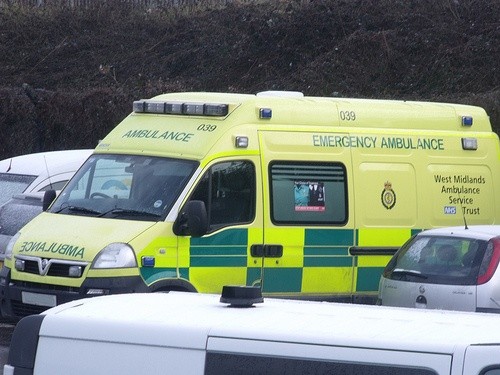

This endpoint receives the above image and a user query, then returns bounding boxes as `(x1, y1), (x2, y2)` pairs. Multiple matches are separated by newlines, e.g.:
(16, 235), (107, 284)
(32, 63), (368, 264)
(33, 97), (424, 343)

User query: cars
(0, 149), (134, 208)
(0, 190), (133, 273)
(376, 224), (500, 314)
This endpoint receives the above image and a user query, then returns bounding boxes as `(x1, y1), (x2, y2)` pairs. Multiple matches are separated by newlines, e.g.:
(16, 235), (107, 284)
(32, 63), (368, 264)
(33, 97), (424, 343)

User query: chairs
(431, 245), (462, 274)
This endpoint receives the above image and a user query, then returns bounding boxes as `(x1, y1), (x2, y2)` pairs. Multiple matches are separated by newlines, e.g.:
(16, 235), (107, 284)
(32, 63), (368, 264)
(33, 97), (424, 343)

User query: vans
(0, 285), (500, 375)
(0, 89), (500, 326)
